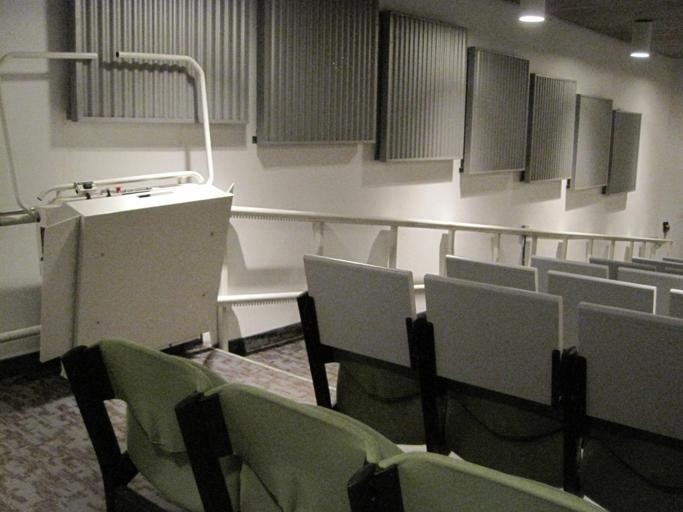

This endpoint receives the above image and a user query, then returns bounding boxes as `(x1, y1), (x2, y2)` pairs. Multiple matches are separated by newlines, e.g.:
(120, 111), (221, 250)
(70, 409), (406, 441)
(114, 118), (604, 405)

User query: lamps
(518, 1), (545, 23)
(629, 18), (654, 59)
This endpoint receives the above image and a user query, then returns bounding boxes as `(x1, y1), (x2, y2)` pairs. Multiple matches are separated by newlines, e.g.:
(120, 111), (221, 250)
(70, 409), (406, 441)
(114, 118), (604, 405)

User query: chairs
(415, 269), (566, 487)
(567, 300), (682, 511)
(172, 381), (403, 511)
(436, 254), (682, 352)
(295, 253), (428, 444)
(60, 335), (240, 510)
(343, 449), (603, 512)
